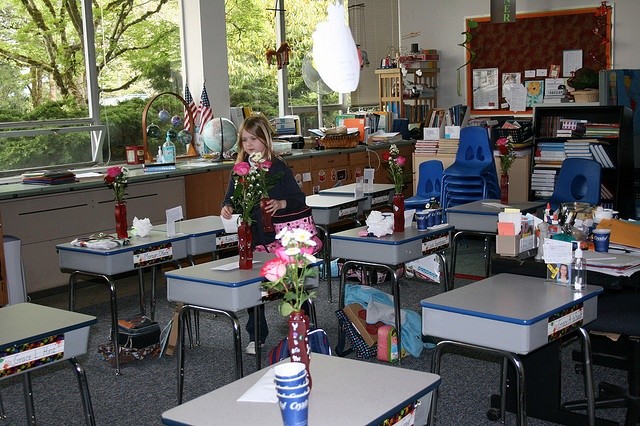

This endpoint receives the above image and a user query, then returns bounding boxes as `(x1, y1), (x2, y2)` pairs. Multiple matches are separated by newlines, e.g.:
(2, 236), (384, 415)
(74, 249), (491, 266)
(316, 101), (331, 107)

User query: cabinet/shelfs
(412, 149), (532, 203)
(185, 140), (415, 266)
(528, 106), (634, 219)
(375, 68), (440, 127)
(1, 176), (185, 296)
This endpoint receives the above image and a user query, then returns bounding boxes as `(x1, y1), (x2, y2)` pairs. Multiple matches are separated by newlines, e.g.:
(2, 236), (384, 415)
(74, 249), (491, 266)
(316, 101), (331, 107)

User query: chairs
(561, 290), (639, 426)
(439, 128), (501, 210)
(549, 158), (602, 205)
(405, 160), (445, 208)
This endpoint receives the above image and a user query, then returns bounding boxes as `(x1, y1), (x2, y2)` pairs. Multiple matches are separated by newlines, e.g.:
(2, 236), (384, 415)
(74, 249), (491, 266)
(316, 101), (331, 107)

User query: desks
(305, 194), (371, 303)
(445, 199), (547, 278)
(0, 302), (98, 426)
(150, 215), (238, 348)
(331, 221), (455, 365)
(163, 251), (322, 404)
(419, 273), (604, 425)
(162, 353), (441, 426)
(319, 181), (396, 227)
(56, 228), (195, 375)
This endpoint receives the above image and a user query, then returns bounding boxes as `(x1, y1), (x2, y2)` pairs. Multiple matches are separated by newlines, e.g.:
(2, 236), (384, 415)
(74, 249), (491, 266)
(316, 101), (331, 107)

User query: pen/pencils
(543, 202), (577, 227)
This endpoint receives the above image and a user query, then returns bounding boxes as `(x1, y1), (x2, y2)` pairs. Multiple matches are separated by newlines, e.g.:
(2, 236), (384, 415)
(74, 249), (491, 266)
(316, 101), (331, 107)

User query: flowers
(102, 167), (129, 202)
(496, 139), (517, 172)
(258, 227), (322, 318)
(229, 153), (273, 224)
(382, 144), (406, 195)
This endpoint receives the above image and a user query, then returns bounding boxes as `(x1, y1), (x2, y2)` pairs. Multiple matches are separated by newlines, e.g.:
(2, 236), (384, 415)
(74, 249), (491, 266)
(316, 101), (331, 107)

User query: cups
(416, 212), (429, 231)
(273, 362), (310, 426)
(592, 229), (612, 253)
(423, 208), (442, 227)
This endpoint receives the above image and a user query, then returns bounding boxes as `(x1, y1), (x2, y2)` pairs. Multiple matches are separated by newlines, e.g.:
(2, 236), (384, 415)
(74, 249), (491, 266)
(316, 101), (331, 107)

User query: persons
(557, 264), (571, 283)
(220, 115), (304, 355)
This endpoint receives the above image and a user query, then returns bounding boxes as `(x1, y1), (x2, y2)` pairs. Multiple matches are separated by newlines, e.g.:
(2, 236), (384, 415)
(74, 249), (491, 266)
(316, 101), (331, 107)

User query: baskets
(317, 132), (360, 149)
(571, 89), (599, 103)
(491, 120), (532, 144)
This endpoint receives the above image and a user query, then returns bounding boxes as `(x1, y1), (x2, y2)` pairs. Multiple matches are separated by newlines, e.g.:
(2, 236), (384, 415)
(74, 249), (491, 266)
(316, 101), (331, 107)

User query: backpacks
(270, 326), (332, 365)
(109, 315), (161, 349)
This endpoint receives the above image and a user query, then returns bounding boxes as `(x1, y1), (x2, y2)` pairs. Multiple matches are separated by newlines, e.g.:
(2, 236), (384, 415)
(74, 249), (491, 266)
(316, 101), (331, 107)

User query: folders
(424, 110), (445, 128)
(446, 104), (467, 126)
(269, 117), (294, 125)
(272, 124), (296, 130)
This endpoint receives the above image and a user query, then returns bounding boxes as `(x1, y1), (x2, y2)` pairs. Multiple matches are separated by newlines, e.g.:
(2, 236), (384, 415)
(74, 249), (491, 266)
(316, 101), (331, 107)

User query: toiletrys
(569, 240), (588, 291)
(163, 131), (177, 164)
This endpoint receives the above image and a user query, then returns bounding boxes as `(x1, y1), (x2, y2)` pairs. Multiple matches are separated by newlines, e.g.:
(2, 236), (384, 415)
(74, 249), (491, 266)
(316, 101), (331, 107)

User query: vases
(392, 193), (406, 234)
(501, 175), (509, 206)
(237, 223), (254, 269)
(114, 203), (127, 237)
(288, 311), (313, 389)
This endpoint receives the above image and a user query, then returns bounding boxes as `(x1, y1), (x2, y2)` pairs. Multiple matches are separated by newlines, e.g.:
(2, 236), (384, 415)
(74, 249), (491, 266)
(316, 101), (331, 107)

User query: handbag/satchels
(377, 325), (410, 361)
(272, 197), (318, 239)
(255, 235), (324, 264)
(334, 309), (377, 360)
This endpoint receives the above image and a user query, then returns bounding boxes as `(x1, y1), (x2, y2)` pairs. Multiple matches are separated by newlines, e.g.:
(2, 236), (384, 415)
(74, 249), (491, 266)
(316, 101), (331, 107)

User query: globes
(201, 117), (238, 163)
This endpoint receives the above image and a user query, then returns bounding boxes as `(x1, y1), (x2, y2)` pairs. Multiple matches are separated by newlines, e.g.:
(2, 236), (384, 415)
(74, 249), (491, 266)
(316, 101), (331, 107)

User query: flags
(182, 85), (198, 132)
(198, 84), (213, 135)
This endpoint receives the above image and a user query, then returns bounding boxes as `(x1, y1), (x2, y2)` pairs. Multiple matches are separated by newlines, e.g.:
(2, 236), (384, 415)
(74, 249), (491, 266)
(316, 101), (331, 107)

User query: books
(564, 140), (593, 160)
(438, 138), (462, 156)
(231, 105), (254, 132)
(19, 169), (103, 185)
(424, 108), (446, 128)
(598, 71), (636, 106)
(270, 120), (295, 133)
(600, 183), (613, 200)
(337, 110), (401, 145)
(589, 143), (615, 168)
(556, 130), (572, 140)
(530, 168), (556, 198)
(414, 138), (438, 154)
(450, 104), (468, 126)
(583, 123), (620, 139)
(532, 142), (567, 169)
